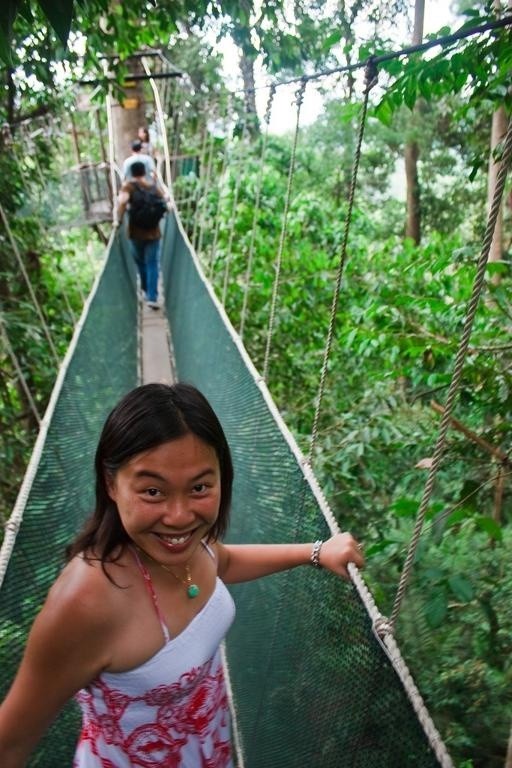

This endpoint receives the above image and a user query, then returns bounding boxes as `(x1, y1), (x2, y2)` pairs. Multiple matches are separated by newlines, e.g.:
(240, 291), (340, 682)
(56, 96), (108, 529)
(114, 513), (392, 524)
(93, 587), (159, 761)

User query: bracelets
(309, 539), (322, 569)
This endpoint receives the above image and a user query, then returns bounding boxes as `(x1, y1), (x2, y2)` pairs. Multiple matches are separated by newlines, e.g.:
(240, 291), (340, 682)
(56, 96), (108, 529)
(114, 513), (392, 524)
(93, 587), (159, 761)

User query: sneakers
(141, 290), (159, 309)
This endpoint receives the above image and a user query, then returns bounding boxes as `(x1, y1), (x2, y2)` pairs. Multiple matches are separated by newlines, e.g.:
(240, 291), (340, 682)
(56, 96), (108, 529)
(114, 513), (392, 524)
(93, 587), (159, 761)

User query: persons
(111, 125), (170, 312)
(0, 384), (364, 768)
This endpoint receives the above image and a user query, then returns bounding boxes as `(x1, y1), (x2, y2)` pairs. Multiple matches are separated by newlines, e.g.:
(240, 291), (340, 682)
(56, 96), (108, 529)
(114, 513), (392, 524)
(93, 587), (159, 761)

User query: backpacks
(128, 181), (166, 229)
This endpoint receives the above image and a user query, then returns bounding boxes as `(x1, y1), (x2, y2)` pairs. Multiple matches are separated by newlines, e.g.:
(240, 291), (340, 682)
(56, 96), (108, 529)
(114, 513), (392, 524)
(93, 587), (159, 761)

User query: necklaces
(134, 545), (200, 599)
